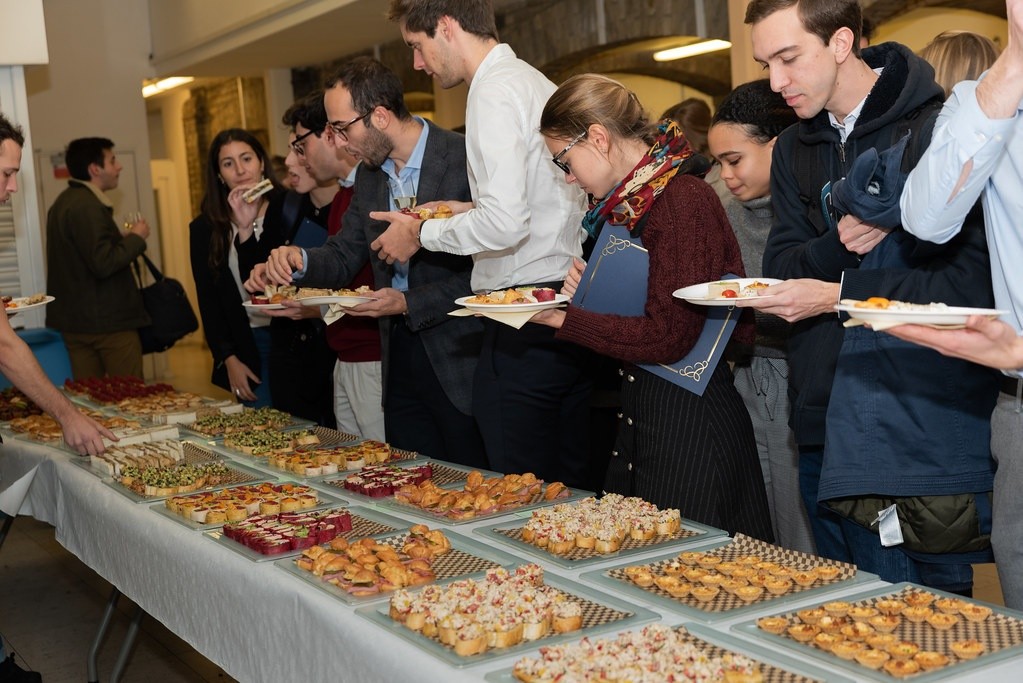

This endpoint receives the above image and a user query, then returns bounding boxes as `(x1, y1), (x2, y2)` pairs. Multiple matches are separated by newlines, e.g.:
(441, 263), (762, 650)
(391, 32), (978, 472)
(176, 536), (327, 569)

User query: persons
(0, 114), (120, 456)
(864, 0), (1023, 608)
(369, 0), (589, 493)
(734, 0), (995, 598)
(228, 86), (385, 444)
(189, 126), (303, 410)
(701, 78), (817, 558)
(45, 137), (152, 381)
(265, 56), (491, 473)
(918, 30), (1000, 102)
(658, 97), (715, 179)
(474, 73), (775, 544)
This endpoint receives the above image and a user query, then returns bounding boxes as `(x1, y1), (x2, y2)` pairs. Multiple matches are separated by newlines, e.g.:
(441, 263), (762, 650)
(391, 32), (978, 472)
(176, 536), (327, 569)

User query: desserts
(707, 280), (769, 298)
(400, 204), (454, 218)
(0, 376), (993, 683)
(249, 285), (374, 306)
(1, 293), (44, 309)
(489, 285), (556, 303)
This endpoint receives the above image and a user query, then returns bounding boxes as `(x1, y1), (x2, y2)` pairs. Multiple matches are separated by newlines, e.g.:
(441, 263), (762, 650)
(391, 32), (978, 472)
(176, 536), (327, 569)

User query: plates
(296, 296), (377, 307)
(672, 277), (785, 305)
(4, 295), (55, 313)
(241, 300), (288, 310)
(453, 293), (570, 312)
(833, 302), (1010, 324)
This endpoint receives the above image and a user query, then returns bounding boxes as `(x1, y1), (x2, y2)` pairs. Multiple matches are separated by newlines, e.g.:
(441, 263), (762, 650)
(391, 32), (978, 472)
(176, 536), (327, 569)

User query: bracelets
(416, 220), (426, 248)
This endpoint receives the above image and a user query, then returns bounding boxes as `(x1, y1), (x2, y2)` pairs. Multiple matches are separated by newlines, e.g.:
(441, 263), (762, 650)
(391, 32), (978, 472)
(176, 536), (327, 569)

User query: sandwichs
(241, 178), (273, 203)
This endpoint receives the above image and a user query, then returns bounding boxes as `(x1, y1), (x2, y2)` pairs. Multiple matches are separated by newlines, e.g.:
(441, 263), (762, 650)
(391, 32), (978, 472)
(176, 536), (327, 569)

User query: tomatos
(722, 289), (737, 298)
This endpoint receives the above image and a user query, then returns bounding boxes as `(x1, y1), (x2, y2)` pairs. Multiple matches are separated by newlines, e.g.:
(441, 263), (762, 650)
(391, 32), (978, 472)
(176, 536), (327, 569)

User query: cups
(123, 211), (141, 233)
(386, 175), (417, 211)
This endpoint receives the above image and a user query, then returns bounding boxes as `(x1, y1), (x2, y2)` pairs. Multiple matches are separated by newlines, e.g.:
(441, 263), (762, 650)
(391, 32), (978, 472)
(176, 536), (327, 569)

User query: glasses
(551, 130), (589, 174)
(326, 104), (388, 142)
(291, 126), (332, 154)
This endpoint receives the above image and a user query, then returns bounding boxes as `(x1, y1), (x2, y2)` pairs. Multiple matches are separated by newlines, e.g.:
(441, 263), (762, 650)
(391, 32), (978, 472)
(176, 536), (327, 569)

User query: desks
(0, 396), (966, 682)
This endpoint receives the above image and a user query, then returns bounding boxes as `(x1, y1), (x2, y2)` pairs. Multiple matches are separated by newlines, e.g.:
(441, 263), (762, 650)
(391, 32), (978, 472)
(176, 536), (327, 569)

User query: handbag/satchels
(132, 249), (199, 356)
